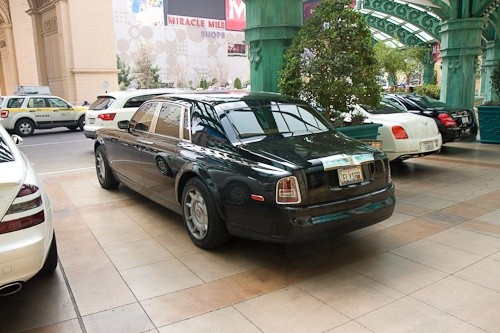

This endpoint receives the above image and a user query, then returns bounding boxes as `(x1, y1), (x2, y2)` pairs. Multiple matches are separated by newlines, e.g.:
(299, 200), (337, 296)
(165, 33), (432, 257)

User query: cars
(0, 125), (59, 297)
(317, 91), (479, 163)
(94, 90), (395, 250)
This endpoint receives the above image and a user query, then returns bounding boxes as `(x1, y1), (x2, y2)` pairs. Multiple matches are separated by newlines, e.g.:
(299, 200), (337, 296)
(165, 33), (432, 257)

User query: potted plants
(277, 0), (383, 140)
(475, 62), (500, 143)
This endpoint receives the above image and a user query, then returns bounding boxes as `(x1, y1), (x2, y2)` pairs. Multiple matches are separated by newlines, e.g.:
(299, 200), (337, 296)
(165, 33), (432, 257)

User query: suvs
(0, 94), (91, 137)
(81, 87), (185, 140)
